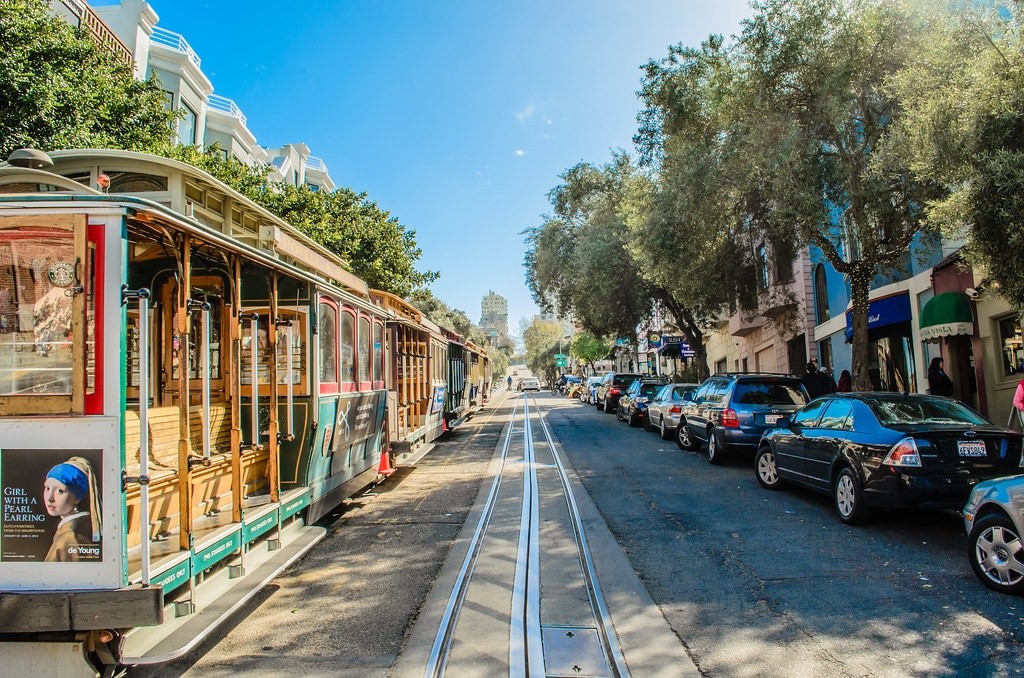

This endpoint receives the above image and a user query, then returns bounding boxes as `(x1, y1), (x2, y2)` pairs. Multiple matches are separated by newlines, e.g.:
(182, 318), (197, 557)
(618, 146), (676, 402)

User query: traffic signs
(554, 354), (568, 358)
(556, 360), (567, 367)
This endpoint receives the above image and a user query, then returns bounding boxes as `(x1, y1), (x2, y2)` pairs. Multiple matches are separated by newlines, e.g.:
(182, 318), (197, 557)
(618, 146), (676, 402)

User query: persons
(43, 456), (102, 561)
(928, 357), (954, 396)
(557, 373), (567, 392)
(801, 359), (851, 399)
(507, 376), (512, 386)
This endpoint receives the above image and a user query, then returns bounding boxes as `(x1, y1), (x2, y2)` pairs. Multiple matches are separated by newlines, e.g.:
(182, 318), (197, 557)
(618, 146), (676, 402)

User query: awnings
(920, 290), (974, 344)
(658, 335), (684, 356)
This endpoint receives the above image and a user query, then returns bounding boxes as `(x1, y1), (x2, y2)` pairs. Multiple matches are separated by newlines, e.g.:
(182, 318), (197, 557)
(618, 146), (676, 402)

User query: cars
(537, 377), (548, 390)
(551, 375), (605, 405)
(754, 391), (1023, 525)
(617, 377), (671, 427)
(643, 382), (702, 440)
(521, 377), (540, 391)
(596, 372), (645, 413)
(675, 372), (812, 465)
(962, 474), (1024, 595)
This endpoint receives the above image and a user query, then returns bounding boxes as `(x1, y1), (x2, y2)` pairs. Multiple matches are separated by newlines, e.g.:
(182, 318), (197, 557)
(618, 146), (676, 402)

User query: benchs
(125, 402), (282, 549)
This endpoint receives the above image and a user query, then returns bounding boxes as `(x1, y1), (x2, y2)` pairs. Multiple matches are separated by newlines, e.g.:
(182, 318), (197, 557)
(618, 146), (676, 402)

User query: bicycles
(507, 380), (512, 390)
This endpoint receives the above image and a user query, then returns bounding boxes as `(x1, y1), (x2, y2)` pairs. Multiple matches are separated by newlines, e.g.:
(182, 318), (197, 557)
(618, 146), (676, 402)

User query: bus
(0, 149), (491, 678)
